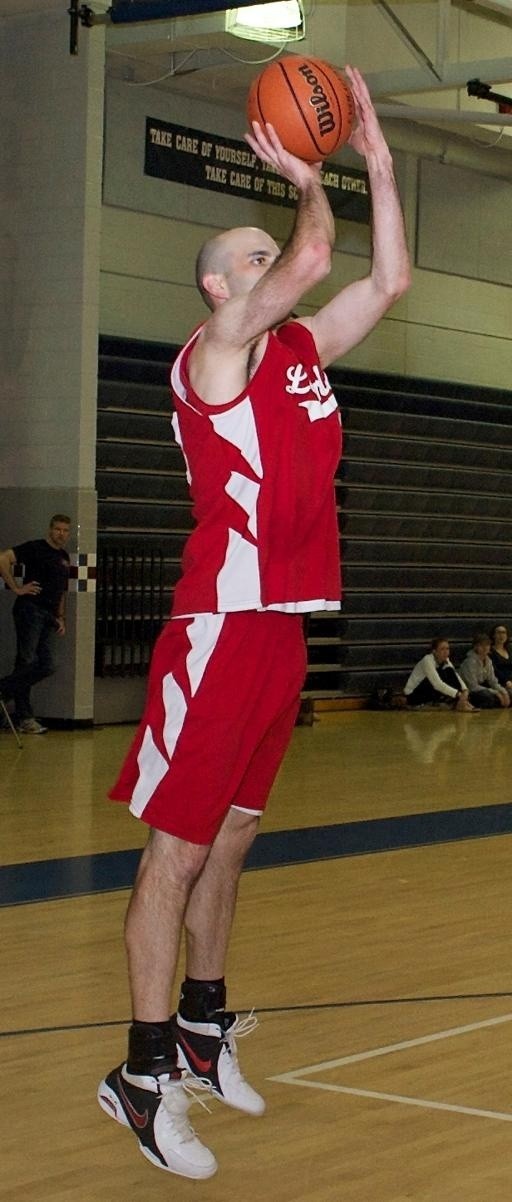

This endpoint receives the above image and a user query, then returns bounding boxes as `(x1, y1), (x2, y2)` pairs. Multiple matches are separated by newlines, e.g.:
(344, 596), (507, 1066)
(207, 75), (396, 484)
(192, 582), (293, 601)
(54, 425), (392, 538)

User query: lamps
(225, 0), (306, 44)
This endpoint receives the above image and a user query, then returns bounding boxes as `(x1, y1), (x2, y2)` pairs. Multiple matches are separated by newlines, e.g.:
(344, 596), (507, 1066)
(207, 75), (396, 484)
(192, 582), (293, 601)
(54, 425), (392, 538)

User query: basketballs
(248, 55), (353, 163)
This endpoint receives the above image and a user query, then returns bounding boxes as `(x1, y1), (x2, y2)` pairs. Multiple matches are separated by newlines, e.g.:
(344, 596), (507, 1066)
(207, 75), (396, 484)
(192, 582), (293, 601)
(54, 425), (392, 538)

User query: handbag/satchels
(369, 687), (407, 711)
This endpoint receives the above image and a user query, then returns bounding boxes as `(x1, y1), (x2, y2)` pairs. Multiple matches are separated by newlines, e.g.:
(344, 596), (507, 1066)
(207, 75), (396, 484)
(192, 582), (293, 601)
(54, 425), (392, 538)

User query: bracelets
(57, 615), (66, 620)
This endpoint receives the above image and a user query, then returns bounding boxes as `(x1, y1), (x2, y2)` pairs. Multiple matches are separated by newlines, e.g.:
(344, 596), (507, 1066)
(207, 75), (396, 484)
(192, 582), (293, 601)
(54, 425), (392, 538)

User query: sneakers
(455, 699), (480, 712)
(16, 717), (48, 734)
(170, 1010), (267, 1116)
(97, 1059), (218, 1180)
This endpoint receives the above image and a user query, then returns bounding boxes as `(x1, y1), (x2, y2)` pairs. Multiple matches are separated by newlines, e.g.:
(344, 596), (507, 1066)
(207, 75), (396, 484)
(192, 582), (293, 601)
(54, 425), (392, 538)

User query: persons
(398, 708), (472, 787)
(0, 513), (72, 737)
(93, 62), (415, 1181)
(401, 636), (479, 712)
(489, 623), (512, 697)
(461, 633), (512, 709)
